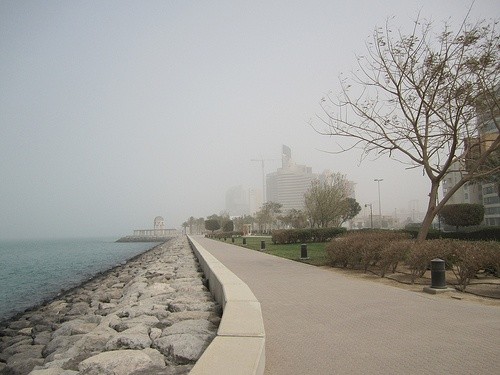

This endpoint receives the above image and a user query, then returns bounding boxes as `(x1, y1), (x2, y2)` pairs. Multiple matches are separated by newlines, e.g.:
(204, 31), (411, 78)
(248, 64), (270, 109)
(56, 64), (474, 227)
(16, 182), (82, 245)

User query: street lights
(374, 177), (385, 226)
(365, 203), (374, 227)
